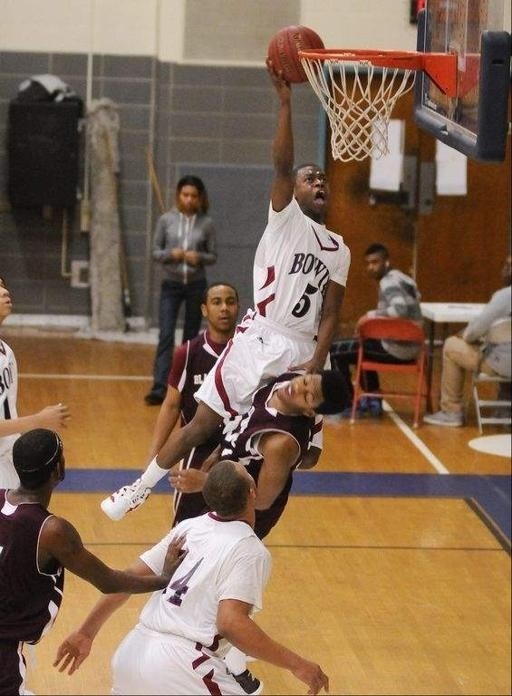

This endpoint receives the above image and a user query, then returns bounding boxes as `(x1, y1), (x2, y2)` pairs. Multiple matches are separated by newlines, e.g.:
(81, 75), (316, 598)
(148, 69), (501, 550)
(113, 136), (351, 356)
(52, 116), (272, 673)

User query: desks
(415, 300), (498, 426)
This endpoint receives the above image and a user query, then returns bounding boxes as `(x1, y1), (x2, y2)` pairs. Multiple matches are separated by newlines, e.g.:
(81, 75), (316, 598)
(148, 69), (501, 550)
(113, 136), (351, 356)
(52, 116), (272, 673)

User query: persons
(96, 55), (355, 523)
(161, 366), (355, 540)
(327, 241), (428, 424)
(50, 459), (330, 696)
(141, 172), (220, 406)
(422, 254), (512, 427)
(147, 277), (242, 518)
(0, 427), (191, 696)
(0, 278), (71, 489)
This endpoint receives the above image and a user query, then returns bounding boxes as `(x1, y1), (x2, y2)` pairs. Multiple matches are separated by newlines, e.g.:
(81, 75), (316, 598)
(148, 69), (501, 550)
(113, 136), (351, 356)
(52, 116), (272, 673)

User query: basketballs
(268, 26), (322, 85)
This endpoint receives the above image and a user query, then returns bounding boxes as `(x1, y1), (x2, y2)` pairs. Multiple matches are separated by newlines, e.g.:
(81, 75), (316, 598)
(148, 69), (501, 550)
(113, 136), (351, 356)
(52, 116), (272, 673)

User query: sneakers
(341, 391), (384, 420)
(489, 408), (512, 431)
(420, 408), (465, 428)
(225, 669), (264, 695)
(142, 381), (168, 407)
(98, 476), (152, 523)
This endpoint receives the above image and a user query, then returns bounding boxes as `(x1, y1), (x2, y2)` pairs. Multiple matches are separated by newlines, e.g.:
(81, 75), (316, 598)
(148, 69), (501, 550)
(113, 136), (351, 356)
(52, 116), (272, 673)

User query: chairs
(469, 340), (512, 440)
(349, 313), (434, 429)
